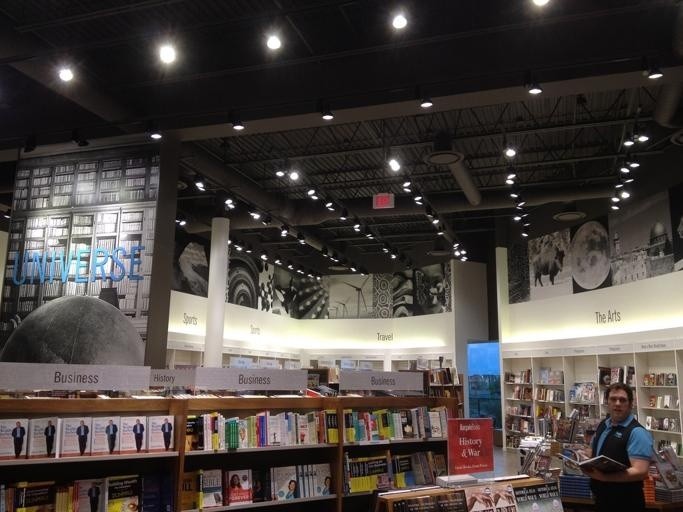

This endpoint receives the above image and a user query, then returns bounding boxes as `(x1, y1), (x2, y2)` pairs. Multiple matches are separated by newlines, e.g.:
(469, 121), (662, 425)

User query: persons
(589, 383), (653, 511)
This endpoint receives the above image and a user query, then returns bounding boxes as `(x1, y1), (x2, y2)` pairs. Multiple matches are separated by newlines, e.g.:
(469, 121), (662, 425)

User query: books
(1, 471), (174, 511)
(1, 416), (174, 459)
(253, 462), (330, 502)
(307, 368), (463, 495)
(182, 468), (253, 511)
(372, 472), (563, 511)
(1, 390), (314, 399)
(185, 410), (339, 451)
(0, 156), (159, 347)
(504, 363), (682, 504)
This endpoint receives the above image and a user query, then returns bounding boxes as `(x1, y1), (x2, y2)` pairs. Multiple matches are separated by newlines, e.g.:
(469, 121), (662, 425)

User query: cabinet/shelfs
(170, 347), (456, 391)
(0, 382), (459, 512)
(512, 432), (562, 479)
(557, 457), (682, 511)
(499, 345), (681, 458)
